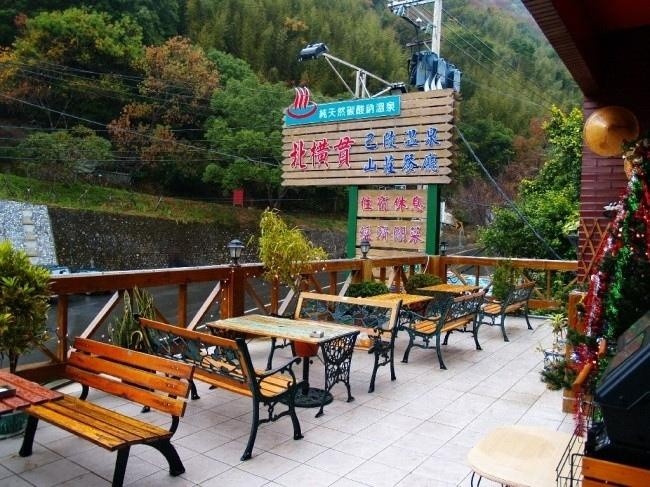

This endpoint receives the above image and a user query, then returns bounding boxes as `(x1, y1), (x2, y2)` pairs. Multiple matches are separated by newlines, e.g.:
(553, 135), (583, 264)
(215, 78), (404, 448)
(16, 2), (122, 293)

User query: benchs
(473, 281), (537, 342)
(266, 292), (403, 393)
(401, 288), (489, 370)
(133, 314), (304, 461)
(18, 336), (196, 487)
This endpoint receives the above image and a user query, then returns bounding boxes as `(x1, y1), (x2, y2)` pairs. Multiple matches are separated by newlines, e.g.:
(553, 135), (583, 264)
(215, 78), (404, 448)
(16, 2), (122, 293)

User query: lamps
(440, 241), (448, 256)
(360, 239), (371, 259)
(227, 239), (244, 266)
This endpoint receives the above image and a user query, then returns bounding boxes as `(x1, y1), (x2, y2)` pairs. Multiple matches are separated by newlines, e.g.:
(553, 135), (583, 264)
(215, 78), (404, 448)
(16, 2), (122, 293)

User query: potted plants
(258, 206), (329, 357)
(404, 272), (442, 316)
(108, 286), (158, 392)
(0, 237), (56, 439)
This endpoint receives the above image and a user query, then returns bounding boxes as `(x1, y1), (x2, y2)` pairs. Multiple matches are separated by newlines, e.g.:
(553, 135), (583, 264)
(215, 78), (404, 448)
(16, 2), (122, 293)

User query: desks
(416, 284), (483, 316)
(366, 292), (435, 330)
(206, 314), (360, 418)
(0, 370), (64, 416)
(468, 425), (584, 487)
